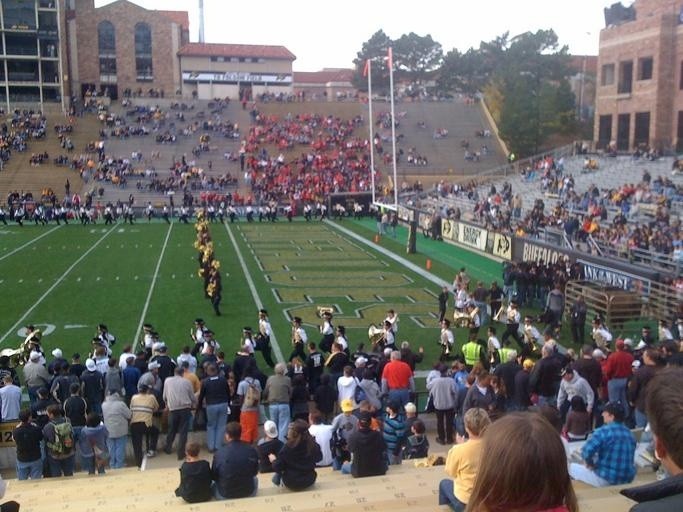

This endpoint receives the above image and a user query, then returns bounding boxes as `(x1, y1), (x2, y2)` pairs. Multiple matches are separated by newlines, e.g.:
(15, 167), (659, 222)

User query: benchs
(0, 94), (373, 210)
(414, 142), (683, 268)
(375, 95), (506, 209)
(0, 412), (659, 512)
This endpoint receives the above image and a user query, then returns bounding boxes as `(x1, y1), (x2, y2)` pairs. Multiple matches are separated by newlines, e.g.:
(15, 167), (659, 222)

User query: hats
(632, 360), (642, 368)
(404, 402), (417, 413)
(359, 411), (371, 422)
(616, 340), (625, 349)
(264, 420), (278, 439)
(598, 400), (626, 419)
(558, 366), (572, 376)
(383, 399), (400, 410)
(1, 342), (250, 392)
(355, 356), (369, 365)
(341, 399), (353, 412)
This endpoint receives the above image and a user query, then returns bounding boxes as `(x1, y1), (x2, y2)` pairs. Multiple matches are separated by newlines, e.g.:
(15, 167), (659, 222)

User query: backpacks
(244, 379), (260, 410)
(46, 418), (75, 456)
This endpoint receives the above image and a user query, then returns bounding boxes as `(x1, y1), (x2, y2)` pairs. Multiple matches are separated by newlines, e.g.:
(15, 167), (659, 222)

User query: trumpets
(444, 334), (453, 355)
(522, 328), (540, 351)
(492, 304), (505, 321)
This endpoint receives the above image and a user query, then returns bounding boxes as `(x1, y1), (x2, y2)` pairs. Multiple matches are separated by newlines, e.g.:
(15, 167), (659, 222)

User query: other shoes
(147, 449), (157, 457)
(436, 438), (445, 444)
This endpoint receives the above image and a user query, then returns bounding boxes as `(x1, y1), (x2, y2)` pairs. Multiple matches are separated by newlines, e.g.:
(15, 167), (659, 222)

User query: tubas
(452, 305), (482, 328)
(1, 329), (42, 368)
(317, 305), (333, 331)
(367, 322), (387, 339)
